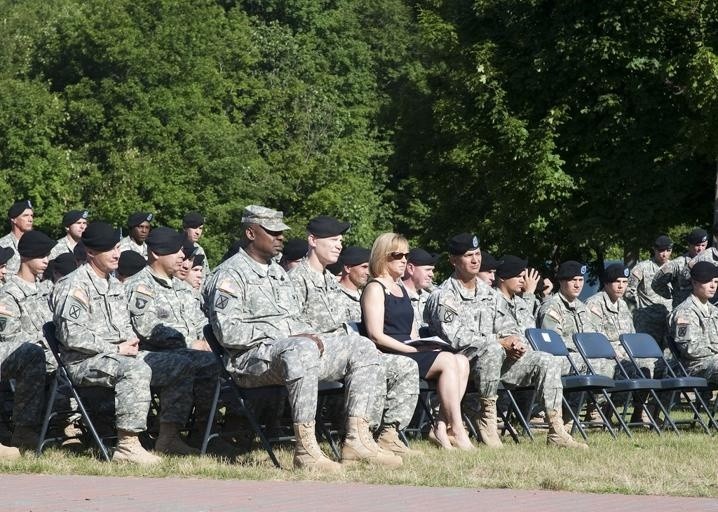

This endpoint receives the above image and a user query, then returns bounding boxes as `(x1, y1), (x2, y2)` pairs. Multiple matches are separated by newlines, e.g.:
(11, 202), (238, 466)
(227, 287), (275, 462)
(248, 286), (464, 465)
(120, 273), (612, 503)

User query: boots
(46, 412), (69, 452)
(342, 417), (403, 465)
(266, 416), (288, 439)
(584, 406), (601, 428)
(59, 414), (83, 449)
(546, 418), (588, 450)
(650, 400), (667, 429)
(293, 420), (342, 472)
(563, 406), (578, 434)
(470, 397), (505, 448)
(602, 402), (616, 430)
(532, 406), (545, 418)
(627, 404), (647, 428)
(377, 428), (422, 458)
(154, 421), (200, 457)
(111, 430), (164, 465)
(0, 417), (11, 438)
(11, 428), (48, 450)
(188, 420), (231, 453)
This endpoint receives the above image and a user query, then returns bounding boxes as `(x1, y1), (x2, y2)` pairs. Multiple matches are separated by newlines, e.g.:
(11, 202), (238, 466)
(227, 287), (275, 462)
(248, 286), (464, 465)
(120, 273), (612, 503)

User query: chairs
(664, 336), (718, 433)
(360, 329), (480, 444)
(200, 324), (342, 468)
(36, 322), (154, 463)
(458, 377), (535, 443)
(525, 327), (631, 441)
(619, 334), (713, 436)
(573, 332), (663, 438)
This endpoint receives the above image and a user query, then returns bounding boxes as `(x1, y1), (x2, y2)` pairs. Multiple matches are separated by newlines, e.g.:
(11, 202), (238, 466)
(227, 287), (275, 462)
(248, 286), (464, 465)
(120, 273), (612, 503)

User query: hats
(406, 248), (440, 265)
(118, 251), (148, 276)
(62, 209), (89, 226)
(689, 261), (717, 283)
(603, 263), (630, 283)
(241, 206), (291, 232)
(128, 213), (153, 229)
(307, 216), (349, 237)
(690, 230), (707, 244)
(0, 246), (15, 266)
(652, 235), (676, 252)
(479, 250), (505, 273)
(192, 254), (204, 268)
(449, 233), (479, 254)
(284, 239), (308, 260)
(18, 231), (58, 256)
(497, 255), (528, 279)
(344, 246), (370, 266)
(8, 200), (35, 218)
(145, 225), (181, 256)
(524, 266), (549, 293)
(183, 212), (204, 229)
(180, 237), (198, 261)
(82, 222), (120, 251)
(327, 255), (344, 276)
(54, 253), (76, 275)
(74, 242), (86, 260)
(558, 261), (587, 280)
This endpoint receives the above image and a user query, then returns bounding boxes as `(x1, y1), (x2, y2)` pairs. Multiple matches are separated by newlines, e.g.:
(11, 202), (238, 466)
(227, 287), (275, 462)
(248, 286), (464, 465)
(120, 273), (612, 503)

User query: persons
(426, 232), (590, 449)
(0, 341), (47, 461)
(477, 251), (505, 287)
(283, 239), (310, 272)
(122, 226), (219, 426)
(359, 232), (475, 450)
(121, 212), (154, 261)
(652, 228), (709, 310)
(521, 264), (554, 320)
(665, 260), (718, 384)
(403, 247), (438, 339)
(492, 255), (589, 433)
(583, 262), (654, 429)
(624, 235), (670, 422)
(51, 222), (201, 464)
(0, 230), (59, 374)
(338, 246), (371, 336)
(182, 213), (211, 277)
(0, 198), (34, 282)
(175, 240), (196, 281)
(49, 209), (88, 260)
(187, 254), (205, 289)
(286, 215), (424, 459)
(673, 222), (718, 306)
(206, 204), (404, 472)
(0, 246), (15, 288)
(533, 260), (615, 429)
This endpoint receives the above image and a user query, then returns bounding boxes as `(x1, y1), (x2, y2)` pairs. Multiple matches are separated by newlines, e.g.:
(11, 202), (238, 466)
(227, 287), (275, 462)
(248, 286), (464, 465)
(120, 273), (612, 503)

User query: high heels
(447, 427), (477, 451)
(428, 425), (455, 451)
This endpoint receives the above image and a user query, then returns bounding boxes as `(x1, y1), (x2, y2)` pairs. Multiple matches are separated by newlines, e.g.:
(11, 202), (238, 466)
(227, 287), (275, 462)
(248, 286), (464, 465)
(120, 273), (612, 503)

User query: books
(404, 336), (451, 349)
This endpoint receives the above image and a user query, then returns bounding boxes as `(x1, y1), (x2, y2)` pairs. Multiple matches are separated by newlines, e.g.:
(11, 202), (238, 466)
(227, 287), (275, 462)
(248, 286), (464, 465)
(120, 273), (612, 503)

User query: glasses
(388, 252), (410, 260)
